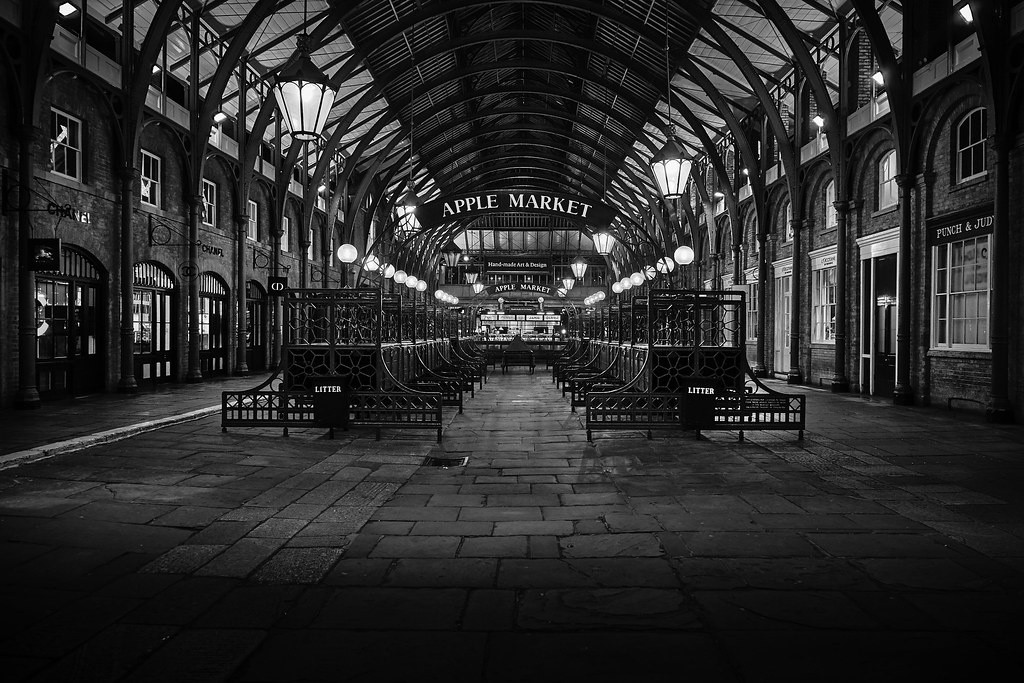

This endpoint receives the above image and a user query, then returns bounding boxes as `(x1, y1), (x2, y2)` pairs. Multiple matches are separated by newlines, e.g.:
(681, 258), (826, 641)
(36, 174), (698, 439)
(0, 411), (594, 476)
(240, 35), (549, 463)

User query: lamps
(275, 0), (695, 304)
